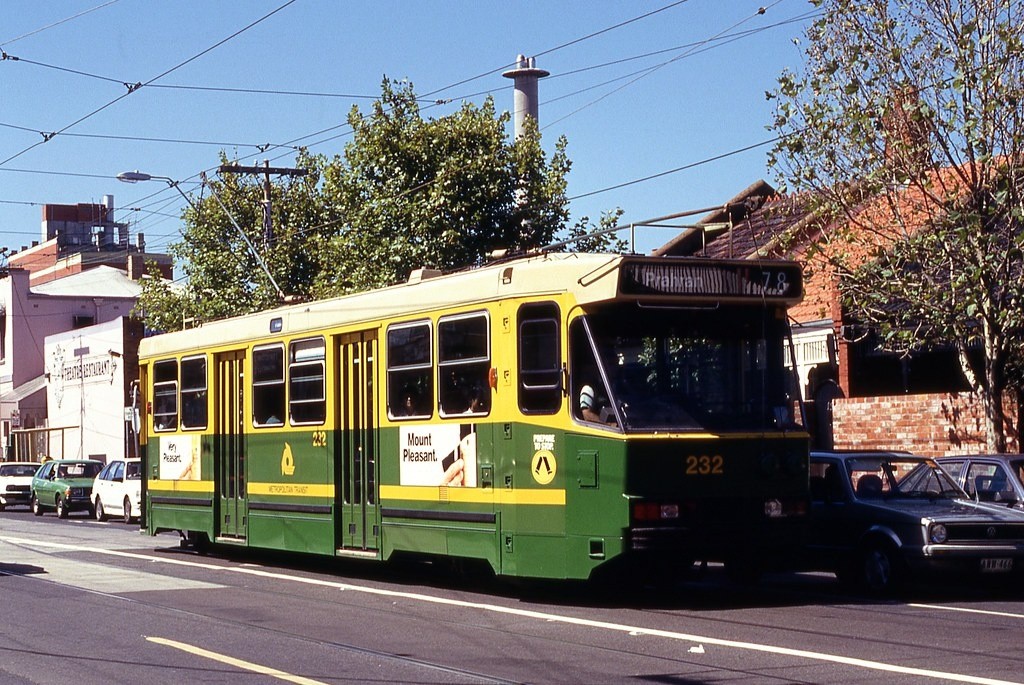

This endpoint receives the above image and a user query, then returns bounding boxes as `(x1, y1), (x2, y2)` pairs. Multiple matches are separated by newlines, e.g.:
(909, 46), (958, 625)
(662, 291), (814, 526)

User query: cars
(0, 461), (43, 513)
(90, 457), (142, 524)
(890, 452), (1024, 510)
(29, 458), (107, 519)
(724, 450), (1023, 600)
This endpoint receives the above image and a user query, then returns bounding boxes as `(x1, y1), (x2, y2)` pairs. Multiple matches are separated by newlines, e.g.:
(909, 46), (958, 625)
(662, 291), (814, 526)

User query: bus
(134, 249), (806, 591)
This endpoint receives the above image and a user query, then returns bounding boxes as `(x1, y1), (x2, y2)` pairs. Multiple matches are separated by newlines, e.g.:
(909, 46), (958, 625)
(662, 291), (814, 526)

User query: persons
(265, 401), (283, 425)
(437, 458), (466, 487)
(49, 464), (66, 481)
(812, 362), (848, 452)
(389, 365), (488, 417)
(578, 350), (637, 427)
